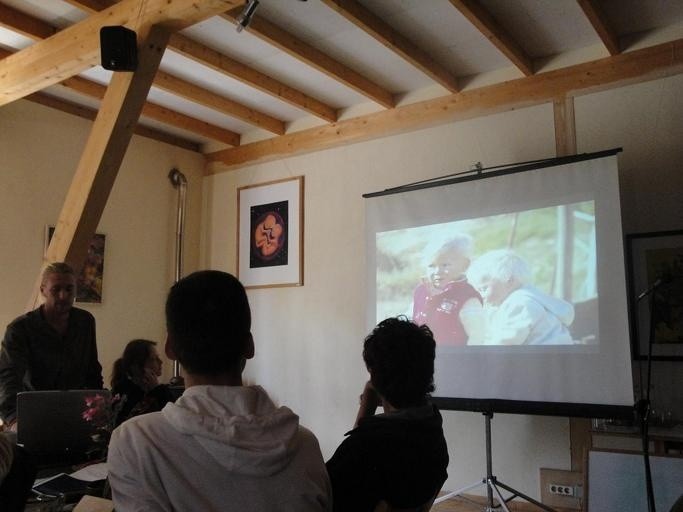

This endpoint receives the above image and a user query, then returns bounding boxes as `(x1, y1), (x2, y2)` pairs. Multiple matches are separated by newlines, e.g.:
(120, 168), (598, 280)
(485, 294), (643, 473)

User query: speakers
(100, 25), (138, 72)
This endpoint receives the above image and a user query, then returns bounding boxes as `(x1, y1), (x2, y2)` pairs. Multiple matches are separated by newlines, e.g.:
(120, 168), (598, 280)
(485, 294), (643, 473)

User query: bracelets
(7, 419), (17, 430)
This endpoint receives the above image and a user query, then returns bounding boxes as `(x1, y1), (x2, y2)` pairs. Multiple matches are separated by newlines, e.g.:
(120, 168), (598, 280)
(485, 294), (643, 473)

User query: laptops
(16, 389), (112, 470)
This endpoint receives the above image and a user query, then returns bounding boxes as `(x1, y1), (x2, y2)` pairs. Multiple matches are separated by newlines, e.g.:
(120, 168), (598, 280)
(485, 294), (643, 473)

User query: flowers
(81, 392), (128, 436)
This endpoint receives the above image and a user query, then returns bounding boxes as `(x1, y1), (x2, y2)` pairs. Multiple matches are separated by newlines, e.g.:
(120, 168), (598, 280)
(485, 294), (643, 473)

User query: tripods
(433, 412), (558, 512)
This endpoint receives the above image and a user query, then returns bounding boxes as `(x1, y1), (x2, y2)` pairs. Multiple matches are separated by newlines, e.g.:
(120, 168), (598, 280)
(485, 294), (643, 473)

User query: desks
(588, 418), (683, 457)
(20, 440), (113, 512)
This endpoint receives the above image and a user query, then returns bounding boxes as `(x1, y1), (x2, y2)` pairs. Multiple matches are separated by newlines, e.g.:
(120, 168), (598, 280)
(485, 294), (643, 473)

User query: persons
(105, 269), (335, 512)
(465, 251), (575, 346)
(110, 340), (173, 430)
(0, 262), (103, 437)
(411, 230), (483, 346)
(325, 314), (449, 512)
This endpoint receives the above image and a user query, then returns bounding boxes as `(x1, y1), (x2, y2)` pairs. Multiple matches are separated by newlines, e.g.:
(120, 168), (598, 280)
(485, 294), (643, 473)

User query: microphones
(636, 278), (662, 303)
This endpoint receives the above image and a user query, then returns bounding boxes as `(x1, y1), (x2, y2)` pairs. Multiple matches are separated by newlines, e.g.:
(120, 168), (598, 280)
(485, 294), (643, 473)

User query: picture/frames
(625, 229), (683, 361)
(237, 175), (304, 290)
(45, 224), (107, 305)
(583, 447), (683, 512)
